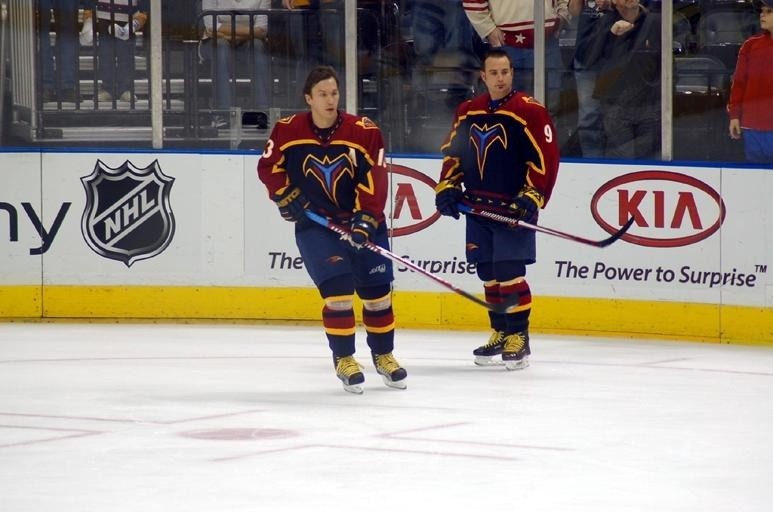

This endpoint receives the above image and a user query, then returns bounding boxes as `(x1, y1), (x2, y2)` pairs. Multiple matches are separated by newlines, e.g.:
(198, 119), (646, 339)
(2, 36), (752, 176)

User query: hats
(753, 1), (773, 8)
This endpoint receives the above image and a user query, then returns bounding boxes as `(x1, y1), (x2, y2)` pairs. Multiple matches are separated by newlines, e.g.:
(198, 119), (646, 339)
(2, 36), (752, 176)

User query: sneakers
(502, 329), (531, 362)
(119, 90), (139, 102)
(474, 329), (508, 356)
(211, 114), (231, 129)
(371, 350), (407, 383)
(91, 90), (116, 103)
(42, 86), (85, 104)
(333, 352), (365, 386)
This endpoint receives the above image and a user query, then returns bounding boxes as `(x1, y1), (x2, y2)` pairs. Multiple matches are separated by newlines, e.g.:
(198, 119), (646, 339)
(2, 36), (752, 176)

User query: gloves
(435, 179), (464, 220)
(510, 186), (545, 221)
(108, 22), (123, 39)
(270, 183), (319, 228)
(120, 19), (139, 41)
(347, 210), (380, 253)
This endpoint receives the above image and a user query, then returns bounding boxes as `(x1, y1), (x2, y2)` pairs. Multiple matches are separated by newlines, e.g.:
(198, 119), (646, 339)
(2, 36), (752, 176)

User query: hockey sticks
(304, 207), (520, 315)
(454, 199), (635, 247)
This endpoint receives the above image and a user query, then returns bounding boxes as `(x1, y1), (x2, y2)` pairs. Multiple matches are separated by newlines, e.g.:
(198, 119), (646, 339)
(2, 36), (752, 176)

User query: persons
(37, 2), (208, 102)
(256, 67), (407, 384)
(197, 0), (661, 162)
(725, 2), (773, 162)
(436, 48), (561, 359)
